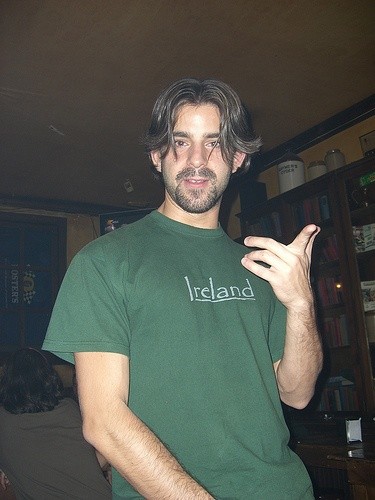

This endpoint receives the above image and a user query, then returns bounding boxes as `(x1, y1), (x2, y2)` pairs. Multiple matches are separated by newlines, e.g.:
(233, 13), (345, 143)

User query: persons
(0, 346), (111, 500)
(42, 79), (324, 500)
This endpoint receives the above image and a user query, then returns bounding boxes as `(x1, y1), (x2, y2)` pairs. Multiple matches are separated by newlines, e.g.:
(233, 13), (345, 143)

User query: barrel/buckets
(325, 148), (346, 172)
(277, 159), (305, 194)
(308, 161), (326, 182)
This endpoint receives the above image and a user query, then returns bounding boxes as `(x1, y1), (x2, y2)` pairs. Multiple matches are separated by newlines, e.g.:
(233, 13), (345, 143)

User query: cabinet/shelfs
(233, 154), (375, 500)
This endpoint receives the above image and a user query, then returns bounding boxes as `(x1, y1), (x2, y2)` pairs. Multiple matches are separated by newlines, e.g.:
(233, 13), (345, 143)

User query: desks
(289, 428), (375, 500)
